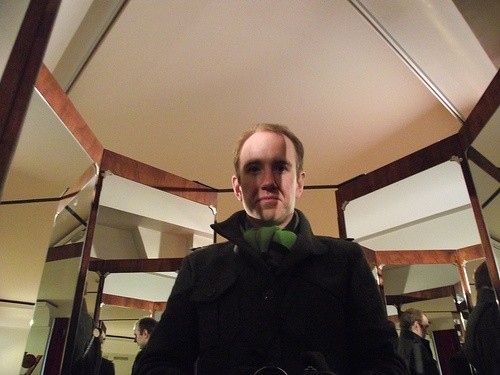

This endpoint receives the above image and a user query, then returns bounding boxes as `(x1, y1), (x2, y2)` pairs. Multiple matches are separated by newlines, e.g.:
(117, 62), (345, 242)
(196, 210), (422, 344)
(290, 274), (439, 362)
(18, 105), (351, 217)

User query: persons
(100, 320), (116, 375)
(139, 123), (422, 375)
(464, 260), (500, 375)
(130, 317), (158, 375)
(398, 308), (440, 375)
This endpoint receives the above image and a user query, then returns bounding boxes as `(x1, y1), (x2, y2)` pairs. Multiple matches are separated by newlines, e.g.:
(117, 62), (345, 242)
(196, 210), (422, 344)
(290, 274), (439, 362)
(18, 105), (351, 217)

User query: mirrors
(0, 62), (500, 375)
(354, 243), (490, 367)
(89, 255), (190, 375)
(19, 243), (82, 375)
(381, 281), (466, 361)
